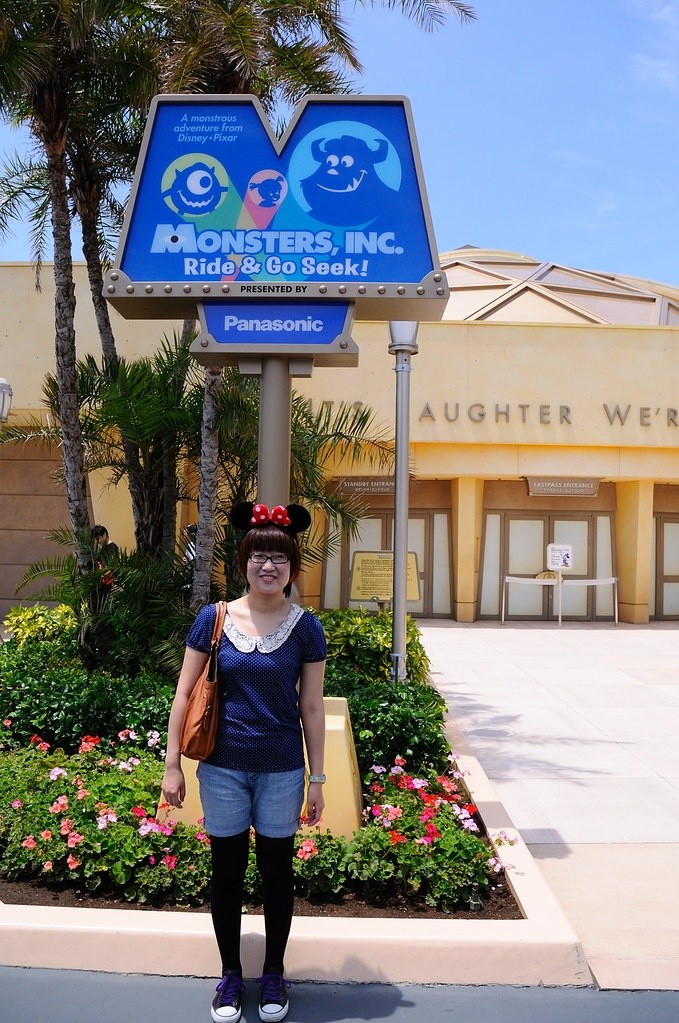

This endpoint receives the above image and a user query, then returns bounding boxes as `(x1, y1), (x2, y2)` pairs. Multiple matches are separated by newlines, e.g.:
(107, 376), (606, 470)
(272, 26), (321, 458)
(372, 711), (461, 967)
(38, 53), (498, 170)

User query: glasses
(249, 553), (289, 564)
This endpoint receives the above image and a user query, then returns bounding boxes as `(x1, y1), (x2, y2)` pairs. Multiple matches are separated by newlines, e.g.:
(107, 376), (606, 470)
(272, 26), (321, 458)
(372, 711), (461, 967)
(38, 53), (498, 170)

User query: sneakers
(258, 973), (291, 1023)
(210, 970), (246, 1023)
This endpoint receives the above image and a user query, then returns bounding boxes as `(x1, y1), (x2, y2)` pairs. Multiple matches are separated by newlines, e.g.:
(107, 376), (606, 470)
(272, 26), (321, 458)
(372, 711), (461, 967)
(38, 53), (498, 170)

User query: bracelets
(308, 774), (326, 785)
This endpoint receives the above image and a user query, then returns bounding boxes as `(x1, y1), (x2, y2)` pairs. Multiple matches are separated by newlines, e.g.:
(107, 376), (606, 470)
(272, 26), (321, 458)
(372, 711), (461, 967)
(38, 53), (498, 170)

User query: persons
(183, 522), (197, 563)
(91, 524), (117, 585)
(160, 525), (328, 1023)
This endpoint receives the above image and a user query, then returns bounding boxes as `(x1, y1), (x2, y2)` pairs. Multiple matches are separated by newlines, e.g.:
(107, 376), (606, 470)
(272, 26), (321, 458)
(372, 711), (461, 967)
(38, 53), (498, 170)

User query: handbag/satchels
(178, 601), (227, 761)
